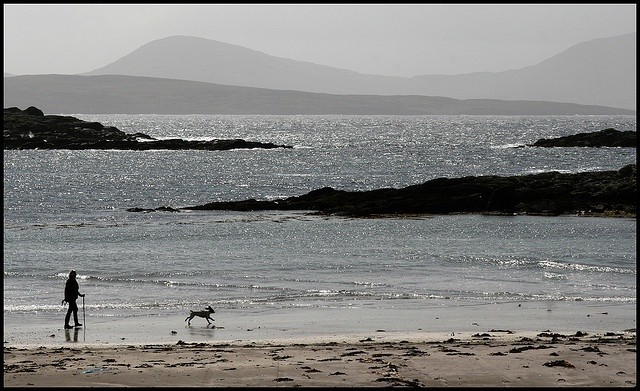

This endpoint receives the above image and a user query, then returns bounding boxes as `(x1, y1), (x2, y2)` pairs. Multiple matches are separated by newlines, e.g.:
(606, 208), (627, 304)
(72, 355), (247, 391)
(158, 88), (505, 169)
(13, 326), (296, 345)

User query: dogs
(185, 306), (215, 326)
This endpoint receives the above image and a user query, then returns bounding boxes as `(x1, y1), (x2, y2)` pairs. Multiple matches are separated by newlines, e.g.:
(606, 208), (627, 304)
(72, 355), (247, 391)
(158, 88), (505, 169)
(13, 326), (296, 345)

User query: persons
(64, 270), (85, 329)
